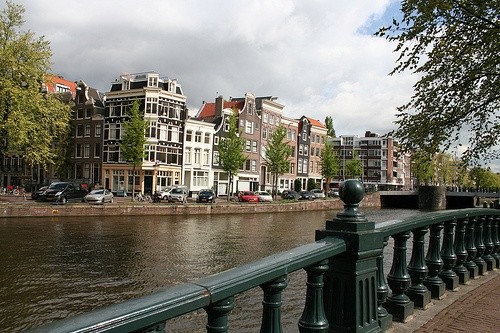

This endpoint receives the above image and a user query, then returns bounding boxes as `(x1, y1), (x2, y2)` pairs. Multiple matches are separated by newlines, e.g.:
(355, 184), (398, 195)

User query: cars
(300, 191), (316, 200)
(31, 185), (49, 200)
(327, 188), (340, 197)
(255, 191), (275, 202)
(84, 189), (114, 205)
(238, 192), (257, 202)
(196, 189), (217, 202)
(170, 188), (187, 201)
(310, 189), (325, 199)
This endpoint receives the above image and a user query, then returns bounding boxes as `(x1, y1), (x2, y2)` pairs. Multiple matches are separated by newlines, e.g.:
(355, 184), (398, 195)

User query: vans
(43, 182), (90, 207)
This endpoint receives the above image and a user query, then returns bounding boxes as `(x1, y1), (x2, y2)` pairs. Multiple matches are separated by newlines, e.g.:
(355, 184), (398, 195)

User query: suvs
(157, 186), (186, 200)
(281, 190), (301, 201)
